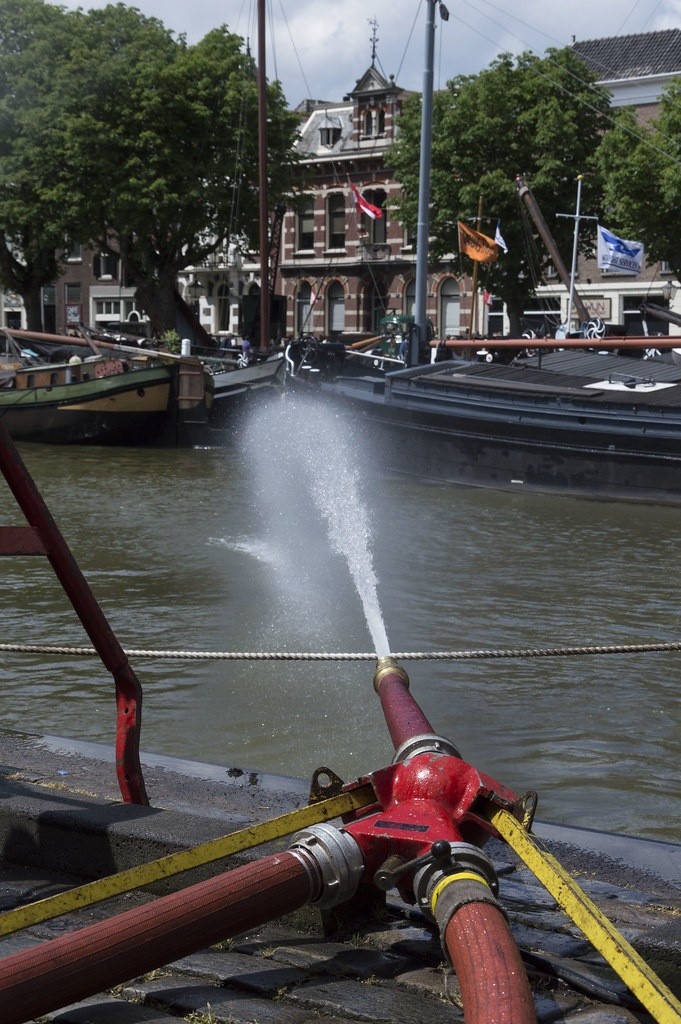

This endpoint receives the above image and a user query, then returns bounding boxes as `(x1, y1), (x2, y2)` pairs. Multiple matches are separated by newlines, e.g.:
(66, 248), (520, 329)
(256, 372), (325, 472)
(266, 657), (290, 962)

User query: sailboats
(54, 0), (341, 436)
(285, 0), (681, 500)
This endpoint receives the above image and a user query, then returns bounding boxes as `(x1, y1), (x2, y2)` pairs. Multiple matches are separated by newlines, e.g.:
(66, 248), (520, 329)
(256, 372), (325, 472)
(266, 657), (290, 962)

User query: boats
(0, 315), (217, 444)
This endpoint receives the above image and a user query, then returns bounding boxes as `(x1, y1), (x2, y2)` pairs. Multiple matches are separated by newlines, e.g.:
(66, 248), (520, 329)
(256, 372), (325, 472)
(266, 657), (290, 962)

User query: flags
(495, 224), (508, 254)
(483, 289), (493, 307)
(458, 220), (499, 263)
(597, 225), (644, 274)
(352, 183), (382, 220)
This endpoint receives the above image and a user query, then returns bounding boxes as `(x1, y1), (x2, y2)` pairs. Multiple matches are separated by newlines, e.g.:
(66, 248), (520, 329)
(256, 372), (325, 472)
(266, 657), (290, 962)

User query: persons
(219, 333), (234, 358)
(400, 338), (409, 361)
(242, 335), (251, 353)
